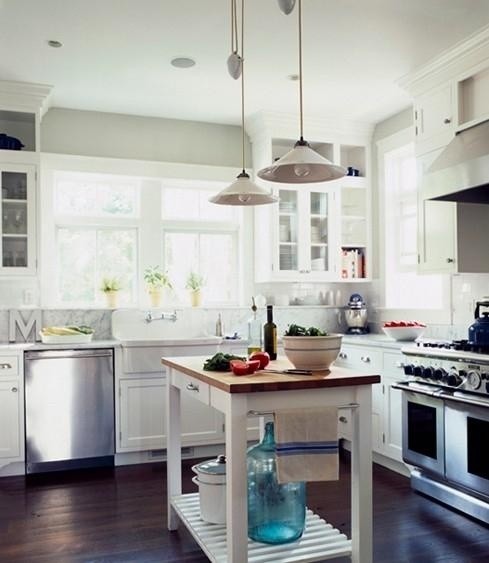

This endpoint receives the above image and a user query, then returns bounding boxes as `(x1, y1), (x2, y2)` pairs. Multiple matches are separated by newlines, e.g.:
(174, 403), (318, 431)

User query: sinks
(112, 307), (223, 373)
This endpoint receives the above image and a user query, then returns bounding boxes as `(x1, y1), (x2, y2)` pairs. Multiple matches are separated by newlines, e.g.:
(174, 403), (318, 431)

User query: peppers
(286, 322), (328, 337)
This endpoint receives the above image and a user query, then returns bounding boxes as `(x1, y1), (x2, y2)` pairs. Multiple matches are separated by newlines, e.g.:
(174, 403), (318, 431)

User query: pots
(0, 133), (25, 151)
(191, 455), (232, 525)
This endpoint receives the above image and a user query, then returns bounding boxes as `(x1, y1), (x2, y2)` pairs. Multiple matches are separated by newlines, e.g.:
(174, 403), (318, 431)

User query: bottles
(215, 312), (225, 337)
(264, 305), (278, 361)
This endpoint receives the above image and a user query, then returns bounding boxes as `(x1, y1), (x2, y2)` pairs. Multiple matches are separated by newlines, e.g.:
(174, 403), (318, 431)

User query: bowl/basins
(279, 332), (343, 372)
(381, 326), (427, 341)
(40, 335), (92, 343)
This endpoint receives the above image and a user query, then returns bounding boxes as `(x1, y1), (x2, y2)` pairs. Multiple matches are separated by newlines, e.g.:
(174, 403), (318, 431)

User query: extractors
(421, 118), (489, 205)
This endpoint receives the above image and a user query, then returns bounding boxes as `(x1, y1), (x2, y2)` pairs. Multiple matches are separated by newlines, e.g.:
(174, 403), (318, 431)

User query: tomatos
(230, 351), (270, 375)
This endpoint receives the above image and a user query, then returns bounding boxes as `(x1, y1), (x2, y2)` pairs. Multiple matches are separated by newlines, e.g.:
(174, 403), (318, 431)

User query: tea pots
(468, 300), (489, 345)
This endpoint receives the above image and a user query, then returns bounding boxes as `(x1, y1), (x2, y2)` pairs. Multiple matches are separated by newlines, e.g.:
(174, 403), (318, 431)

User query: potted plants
(183, 270), (206, 306)
(142, 262), (174, 308)
(100, 274), (124, 309)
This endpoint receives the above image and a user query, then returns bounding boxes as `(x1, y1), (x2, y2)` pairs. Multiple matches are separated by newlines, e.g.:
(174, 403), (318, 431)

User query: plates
(279, 252), (296, 270)
(280, 202), (294, 212)
(312, 258), (325, 270)
(278, 224), (289, 242)
(310, 226), (321, 243)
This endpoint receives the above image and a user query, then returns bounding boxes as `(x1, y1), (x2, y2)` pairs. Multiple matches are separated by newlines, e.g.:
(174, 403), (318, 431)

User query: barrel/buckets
(248, 422), (306, 543)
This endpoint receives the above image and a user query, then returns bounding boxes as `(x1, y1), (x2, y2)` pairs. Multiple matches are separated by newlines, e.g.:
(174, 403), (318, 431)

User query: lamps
(255, 0), (347, 184)
(204, 0), (280, 209)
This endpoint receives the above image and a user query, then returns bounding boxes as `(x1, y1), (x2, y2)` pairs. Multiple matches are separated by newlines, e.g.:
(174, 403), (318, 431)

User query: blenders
(342, 291), (370, 335)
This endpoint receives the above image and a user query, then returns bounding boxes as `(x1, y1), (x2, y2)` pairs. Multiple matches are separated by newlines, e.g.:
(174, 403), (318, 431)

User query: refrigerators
(22, 348), (116, 480)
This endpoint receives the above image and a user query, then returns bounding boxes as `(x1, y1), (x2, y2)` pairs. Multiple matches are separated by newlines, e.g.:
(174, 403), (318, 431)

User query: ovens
(390, 378), (488, 525)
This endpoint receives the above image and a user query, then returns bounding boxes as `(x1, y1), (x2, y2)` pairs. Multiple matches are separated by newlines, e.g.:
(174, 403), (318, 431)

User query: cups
(348, 166), (359, 177)
(2, 250), (25, 266)
(247, 321), (262, 355)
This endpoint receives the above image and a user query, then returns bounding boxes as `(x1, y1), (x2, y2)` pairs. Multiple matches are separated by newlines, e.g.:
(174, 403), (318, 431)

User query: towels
(268, 404), (343, 484)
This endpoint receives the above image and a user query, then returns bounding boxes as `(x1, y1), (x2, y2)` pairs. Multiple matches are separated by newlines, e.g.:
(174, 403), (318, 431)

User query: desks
(160, 353), (382, 563)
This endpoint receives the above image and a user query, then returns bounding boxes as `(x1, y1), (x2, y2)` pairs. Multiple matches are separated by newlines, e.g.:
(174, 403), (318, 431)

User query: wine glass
(1, 209), (23, 234)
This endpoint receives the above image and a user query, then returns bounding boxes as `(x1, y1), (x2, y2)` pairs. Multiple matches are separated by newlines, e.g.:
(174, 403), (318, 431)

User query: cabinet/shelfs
(414, 77), (489, 274)
(251, 110), (342, 286)
(336, 339), (405, 464)
(0, 348), (27, 464)
(1, 103), (40, 280)
(342, 130), (377, 286)
(113, 341), (266, 457)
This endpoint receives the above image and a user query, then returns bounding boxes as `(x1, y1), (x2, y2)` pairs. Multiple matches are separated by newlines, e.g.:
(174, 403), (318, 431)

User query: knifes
(261, 368), (314, 376)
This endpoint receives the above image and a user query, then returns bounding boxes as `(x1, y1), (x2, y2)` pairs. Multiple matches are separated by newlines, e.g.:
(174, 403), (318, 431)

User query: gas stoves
(397, 338), (489, 396)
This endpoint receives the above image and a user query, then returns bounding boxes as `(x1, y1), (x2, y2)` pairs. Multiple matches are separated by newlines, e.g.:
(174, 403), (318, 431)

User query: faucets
(147, 309), (155, 325)
(171, 309), (180, 322)
